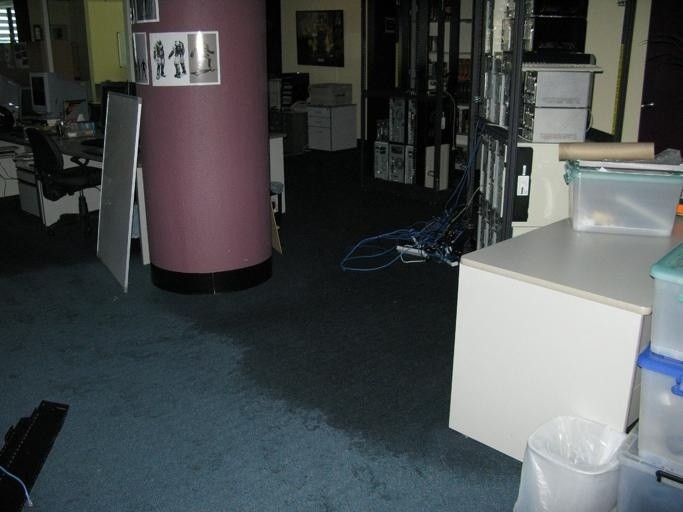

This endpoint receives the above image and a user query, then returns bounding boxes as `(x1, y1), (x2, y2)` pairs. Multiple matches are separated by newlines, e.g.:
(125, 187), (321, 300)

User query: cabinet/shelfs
(449, 220), (683, 478)
(295, 102), (357, 152)
(13, 152), (45, 220)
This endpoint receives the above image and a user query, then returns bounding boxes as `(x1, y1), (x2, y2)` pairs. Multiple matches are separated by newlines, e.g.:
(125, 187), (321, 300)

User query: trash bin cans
(527, 415), (628, 512)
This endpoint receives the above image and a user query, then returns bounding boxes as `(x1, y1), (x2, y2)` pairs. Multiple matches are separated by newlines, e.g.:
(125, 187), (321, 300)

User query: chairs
(27, 127), (102, 239)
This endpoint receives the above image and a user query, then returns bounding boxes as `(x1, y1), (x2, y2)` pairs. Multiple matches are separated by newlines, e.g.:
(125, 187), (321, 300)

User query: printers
(311, 83), (352, 106)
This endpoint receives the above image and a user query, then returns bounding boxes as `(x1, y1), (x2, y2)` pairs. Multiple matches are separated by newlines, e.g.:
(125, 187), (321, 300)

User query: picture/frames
(294, 8), (346, 69)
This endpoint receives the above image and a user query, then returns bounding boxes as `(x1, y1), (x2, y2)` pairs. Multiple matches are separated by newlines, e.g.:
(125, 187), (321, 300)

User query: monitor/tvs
(102, 81), (135, 126)
(29, 72), (88, 120)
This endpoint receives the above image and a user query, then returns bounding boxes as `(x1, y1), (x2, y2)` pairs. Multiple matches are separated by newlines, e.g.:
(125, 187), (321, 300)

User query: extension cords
(396, 243), (430, 258)
(436, 249), (458, 267)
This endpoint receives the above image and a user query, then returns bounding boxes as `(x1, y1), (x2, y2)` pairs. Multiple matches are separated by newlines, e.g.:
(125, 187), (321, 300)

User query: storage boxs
(563, 158), (682, 236)
(650, 240), (682, 362)
(614, 421), (682, 512)
(635, 346), (683, 470)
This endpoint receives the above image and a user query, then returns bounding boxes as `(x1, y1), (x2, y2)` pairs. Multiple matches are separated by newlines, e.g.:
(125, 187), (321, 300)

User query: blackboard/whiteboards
(96, 92), (142, 292)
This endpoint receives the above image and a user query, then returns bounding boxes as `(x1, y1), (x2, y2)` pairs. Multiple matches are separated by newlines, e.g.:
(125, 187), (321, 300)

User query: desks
(0, 116), (152, 265)
